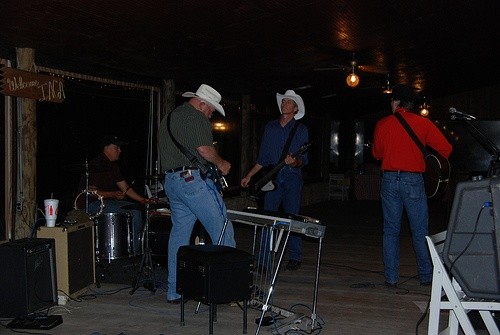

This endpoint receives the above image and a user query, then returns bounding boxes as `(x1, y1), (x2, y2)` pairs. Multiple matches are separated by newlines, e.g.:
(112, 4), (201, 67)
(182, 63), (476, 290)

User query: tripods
(130, 203), (157, 296)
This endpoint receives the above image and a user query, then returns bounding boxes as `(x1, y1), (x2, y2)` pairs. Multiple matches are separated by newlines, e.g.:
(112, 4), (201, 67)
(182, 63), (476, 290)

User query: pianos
(156, 202), (326, 335)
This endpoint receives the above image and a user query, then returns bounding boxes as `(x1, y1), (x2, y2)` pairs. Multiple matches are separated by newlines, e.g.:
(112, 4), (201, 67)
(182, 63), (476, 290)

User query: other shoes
(420, 281), (431, 286)
(167, 297), (188, 304)
(252, 289), (267, 299)
(284, 259), (302, 271)
(384, 281), (397, 288)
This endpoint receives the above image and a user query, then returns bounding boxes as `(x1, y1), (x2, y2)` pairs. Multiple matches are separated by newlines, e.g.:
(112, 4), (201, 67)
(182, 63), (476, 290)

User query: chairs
(425, 231), (500, 335)
(329, 174), (350, 199)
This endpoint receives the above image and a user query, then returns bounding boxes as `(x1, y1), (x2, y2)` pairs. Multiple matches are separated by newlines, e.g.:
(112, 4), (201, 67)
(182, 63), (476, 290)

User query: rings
(286, 160), (288, 162)
(119, 194), (120, 196)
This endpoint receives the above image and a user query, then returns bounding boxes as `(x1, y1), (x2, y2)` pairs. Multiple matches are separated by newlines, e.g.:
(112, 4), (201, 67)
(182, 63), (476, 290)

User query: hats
(276, 89), (306, 121)
(182, 83), (226, 117)
(102, 135), (122, 147)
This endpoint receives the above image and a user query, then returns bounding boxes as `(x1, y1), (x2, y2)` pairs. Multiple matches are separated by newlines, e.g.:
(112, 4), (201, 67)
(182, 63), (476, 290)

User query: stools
(176, 245), (254, 335)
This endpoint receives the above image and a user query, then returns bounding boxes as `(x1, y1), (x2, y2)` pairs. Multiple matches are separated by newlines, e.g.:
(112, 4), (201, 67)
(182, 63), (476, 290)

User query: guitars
(241, 142), (313, 201)
(423, 153), (451, 199)
(206, 140), (228, 196)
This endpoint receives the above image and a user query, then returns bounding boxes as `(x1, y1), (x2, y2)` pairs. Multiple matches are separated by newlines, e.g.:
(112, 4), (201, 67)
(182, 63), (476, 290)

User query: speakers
(36, 221), (95, 300)
(444, 179), (500, 301)
(0, 237), (57, 320)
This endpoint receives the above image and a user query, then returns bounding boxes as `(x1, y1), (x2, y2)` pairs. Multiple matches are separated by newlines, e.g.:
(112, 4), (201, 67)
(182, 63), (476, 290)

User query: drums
(92, 210), (136, 261)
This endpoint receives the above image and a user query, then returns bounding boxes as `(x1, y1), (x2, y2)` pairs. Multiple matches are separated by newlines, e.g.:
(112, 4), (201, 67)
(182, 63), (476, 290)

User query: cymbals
(120, 203), (170, 211)
(143, 175), (165, 180)
(74, 188), (106, 219)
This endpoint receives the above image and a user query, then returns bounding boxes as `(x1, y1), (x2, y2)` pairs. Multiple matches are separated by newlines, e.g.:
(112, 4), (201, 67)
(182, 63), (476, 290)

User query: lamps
(346, 61), (359, 88)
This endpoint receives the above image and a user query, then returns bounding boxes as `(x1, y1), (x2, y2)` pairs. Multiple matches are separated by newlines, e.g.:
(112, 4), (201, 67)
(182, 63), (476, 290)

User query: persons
(77, 135), (163, 270)
(241, 89), (311, 273)
(160, 84), (270, 307)
(371, 84), (453, 287)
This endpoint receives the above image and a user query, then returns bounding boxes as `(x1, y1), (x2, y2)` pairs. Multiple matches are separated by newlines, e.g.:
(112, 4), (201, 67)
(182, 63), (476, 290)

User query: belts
(384, 169), (424, 175)
(167, 166), (198, 173)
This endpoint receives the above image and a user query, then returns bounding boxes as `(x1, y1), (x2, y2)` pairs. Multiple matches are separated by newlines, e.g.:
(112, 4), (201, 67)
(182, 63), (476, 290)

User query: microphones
(449, 107), (476, 120)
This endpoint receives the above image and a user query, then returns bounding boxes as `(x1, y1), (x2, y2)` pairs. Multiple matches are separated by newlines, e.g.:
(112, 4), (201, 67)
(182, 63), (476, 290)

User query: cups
(44, 199), (59, 228)
